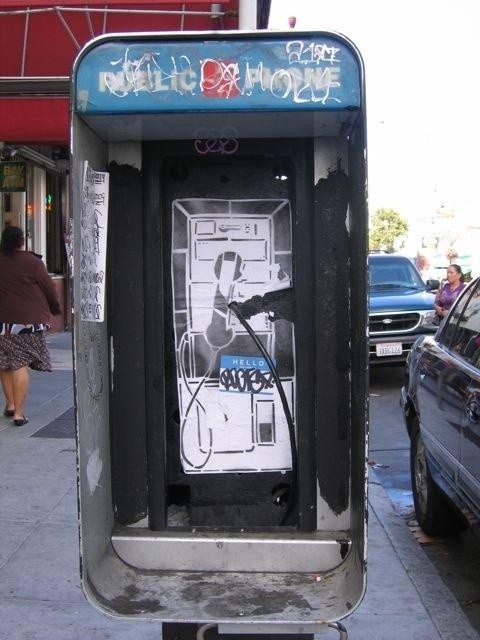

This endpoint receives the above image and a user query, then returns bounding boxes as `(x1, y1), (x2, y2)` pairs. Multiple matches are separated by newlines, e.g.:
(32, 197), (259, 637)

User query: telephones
(205, 252), (243, 348)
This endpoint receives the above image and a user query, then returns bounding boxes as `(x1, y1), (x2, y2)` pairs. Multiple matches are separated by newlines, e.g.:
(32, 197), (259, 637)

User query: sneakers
(3, 409), (28, 426)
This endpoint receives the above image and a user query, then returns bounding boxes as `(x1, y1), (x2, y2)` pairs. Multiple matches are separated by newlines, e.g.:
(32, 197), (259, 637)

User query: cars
(368, 251), (447, 374)
(397, 276), (480, 548)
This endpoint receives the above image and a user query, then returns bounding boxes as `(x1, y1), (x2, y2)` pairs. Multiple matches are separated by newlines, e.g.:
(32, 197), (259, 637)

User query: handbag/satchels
(432, 314), (440, 326)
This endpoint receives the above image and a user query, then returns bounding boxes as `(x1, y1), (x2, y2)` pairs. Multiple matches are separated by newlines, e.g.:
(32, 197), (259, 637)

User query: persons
(0, 226), (62, 427)
(433, 264), (468, 326)
